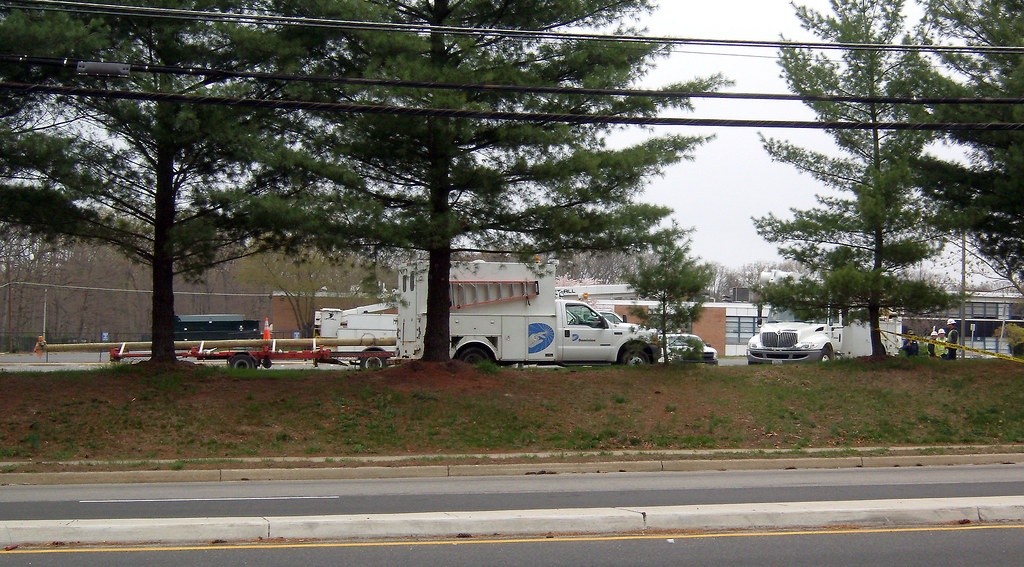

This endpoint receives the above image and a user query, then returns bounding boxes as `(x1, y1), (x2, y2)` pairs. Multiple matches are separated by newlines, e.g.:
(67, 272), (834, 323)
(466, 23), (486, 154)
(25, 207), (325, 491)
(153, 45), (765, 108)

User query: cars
(657, 334), (719, 365)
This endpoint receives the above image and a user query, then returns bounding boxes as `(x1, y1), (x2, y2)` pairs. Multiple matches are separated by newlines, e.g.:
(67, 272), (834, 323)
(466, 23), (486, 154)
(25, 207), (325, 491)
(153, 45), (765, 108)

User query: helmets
(947, 319), (956, 325)
(931, 331), (938, 336)
(938, 329), (946, 334)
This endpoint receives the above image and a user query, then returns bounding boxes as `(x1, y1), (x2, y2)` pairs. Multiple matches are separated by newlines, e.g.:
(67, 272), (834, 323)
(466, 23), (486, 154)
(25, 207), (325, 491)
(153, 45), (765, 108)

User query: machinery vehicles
(746, 268), (903, 367)
(167, 261), (664, 371)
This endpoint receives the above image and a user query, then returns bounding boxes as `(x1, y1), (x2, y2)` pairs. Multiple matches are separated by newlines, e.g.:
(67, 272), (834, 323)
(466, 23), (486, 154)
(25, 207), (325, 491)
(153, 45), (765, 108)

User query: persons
(945, 318), (961, 360)
(927, 331), (938, 357)
(902, 329), (919, 357)
(935, 328), (951, 359)
